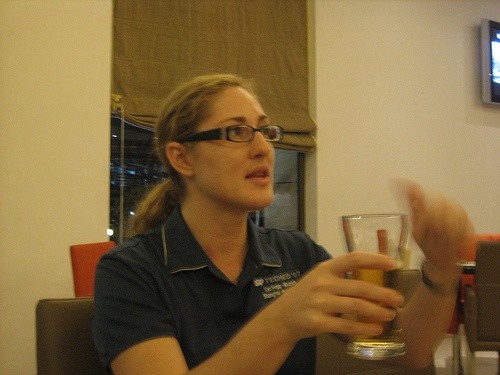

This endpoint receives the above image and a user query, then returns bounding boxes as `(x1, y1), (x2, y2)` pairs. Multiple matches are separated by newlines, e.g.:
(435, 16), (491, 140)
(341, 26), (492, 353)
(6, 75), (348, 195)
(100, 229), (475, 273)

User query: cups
(342, 214), (411, 364)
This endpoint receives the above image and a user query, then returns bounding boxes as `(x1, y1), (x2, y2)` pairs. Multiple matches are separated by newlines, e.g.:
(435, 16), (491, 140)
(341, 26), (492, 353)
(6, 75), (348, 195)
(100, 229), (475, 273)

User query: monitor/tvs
(480, 17), (500, 105)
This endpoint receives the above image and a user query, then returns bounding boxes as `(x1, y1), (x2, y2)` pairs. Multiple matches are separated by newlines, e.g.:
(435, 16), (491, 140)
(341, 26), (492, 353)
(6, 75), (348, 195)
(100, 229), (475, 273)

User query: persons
(91, 74), (471, 375)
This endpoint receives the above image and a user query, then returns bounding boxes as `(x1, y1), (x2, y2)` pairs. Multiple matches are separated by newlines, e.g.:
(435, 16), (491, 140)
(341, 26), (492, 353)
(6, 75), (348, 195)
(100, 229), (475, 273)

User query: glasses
(178, 124), (284, 143)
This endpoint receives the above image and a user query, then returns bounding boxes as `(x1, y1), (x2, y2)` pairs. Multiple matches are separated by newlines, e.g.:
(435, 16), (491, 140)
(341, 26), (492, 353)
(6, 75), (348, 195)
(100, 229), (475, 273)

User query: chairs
(453, 240), (500, 375)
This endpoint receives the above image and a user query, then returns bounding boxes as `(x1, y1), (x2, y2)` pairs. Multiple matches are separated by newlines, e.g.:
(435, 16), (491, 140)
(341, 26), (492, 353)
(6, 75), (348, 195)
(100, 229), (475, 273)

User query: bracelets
(421, 260), (455, 294)
